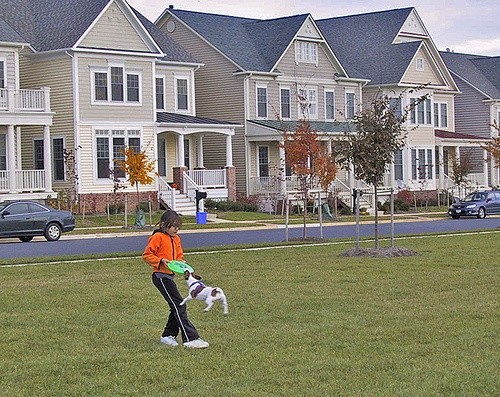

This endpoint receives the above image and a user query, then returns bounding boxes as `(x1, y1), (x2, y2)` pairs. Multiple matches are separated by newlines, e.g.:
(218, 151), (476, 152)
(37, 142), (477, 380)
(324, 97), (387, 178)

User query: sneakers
(183, 338), (209, 349)
(160, 334), (178, 347)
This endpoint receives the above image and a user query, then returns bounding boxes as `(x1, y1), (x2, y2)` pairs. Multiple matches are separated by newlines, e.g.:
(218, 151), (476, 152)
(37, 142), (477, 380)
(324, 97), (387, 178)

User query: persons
(142, 210), (209, 348)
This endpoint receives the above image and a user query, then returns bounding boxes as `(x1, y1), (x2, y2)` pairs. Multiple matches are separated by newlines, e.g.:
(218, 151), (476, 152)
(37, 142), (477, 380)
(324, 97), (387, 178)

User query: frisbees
(167, 260), (195, 275)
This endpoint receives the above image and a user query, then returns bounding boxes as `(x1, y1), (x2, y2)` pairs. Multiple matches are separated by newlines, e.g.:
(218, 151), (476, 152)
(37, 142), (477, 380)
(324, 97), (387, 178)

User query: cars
(447, 190), (500, 219)
(0, 200), (75, 241)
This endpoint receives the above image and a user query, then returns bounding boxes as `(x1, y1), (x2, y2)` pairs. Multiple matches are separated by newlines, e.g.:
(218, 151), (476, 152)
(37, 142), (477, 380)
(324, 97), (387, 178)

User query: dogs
(180, 268), (231, 318)
